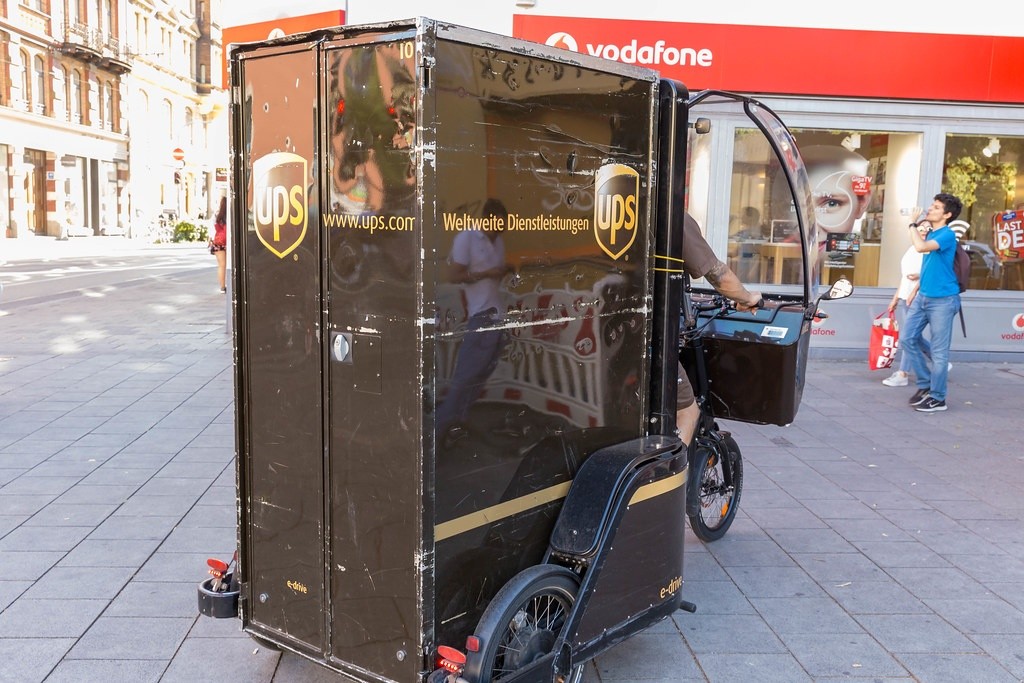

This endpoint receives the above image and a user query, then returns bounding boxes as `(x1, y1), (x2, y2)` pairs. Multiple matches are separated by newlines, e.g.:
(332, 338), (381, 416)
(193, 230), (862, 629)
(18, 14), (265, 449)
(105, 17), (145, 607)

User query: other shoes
(220, 287), (226, 293)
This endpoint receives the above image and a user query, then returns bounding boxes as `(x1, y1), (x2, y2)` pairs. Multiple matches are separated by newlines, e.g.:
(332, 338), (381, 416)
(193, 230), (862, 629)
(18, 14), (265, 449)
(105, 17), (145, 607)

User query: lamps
(982, 137), (1002, 159)
(841, 133), (861, 154)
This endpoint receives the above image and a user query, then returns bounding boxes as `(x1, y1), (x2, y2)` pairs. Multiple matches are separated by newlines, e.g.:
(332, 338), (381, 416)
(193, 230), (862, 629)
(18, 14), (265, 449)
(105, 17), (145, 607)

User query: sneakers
(908, 387), (931, 405)
(882, 370), (908, 386)
(948, 362), (953, 371)
(915, 397), (947, 412)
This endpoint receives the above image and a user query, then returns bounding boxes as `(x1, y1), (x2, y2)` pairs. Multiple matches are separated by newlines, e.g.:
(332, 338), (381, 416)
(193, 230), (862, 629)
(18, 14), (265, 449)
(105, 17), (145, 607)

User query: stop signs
(173, 148), (184, 160)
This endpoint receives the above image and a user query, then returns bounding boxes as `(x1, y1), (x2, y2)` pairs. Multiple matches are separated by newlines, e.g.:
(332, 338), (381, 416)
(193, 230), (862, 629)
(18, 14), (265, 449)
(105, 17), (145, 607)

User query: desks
(728, 239), (881, 286)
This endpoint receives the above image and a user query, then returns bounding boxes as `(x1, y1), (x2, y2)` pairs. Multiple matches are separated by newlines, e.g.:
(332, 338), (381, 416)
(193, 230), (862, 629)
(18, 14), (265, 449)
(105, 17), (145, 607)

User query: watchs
(909, 223), (917, 228)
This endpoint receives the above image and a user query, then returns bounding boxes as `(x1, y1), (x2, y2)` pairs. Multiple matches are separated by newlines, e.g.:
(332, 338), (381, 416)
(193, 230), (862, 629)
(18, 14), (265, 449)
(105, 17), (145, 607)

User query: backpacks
(926, 230), (971, 293)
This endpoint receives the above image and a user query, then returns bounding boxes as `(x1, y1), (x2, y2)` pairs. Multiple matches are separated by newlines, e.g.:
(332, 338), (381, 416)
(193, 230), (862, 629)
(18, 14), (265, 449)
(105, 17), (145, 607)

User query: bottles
(901, 207), (928, 217)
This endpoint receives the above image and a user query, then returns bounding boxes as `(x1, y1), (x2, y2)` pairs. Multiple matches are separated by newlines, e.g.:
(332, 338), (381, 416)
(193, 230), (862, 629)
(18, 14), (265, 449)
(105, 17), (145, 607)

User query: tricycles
(198, 17), (853, 682)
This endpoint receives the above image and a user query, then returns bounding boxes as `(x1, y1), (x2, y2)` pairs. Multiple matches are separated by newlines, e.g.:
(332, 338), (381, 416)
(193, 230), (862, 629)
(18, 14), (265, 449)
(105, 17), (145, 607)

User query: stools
(1001, 258), (1024, 291)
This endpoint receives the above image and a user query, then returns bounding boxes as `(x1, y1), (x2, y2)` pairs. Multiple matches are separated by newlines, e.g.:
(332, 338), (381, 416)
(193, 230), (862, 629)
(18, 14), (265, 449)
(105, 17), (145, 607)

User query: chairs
(961, 249), (1014, 293)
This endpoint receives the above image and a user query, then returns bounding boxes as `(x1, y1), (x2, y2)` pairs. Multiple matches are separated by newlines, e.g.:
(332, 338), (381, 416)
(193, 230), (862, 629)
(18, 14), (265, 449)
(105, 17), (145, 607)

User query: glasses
(916, 226), (933, 232)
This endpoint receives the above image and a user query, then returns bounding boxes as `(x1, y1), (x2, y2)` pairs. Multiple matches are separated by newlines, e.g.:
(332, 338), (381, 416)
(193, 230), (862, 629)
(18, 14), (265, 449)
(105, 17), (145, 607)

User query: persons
(208, 197), (227, 293)
(436, 198), (517, 440)
(900, 193), (962, 411)
(677, 210), (762, 446)
(883, 219), (952, 387)
(808, 166), (874, 257)
(728, 206), (762, 284)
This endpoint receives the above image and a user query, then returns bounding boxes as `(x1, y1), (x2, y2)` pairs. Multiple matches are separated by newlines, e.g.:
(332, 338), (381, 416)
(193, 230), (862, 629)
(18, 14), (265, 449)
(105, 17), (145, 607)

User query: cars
(216, 167), (228, 181)
(958, 240), (1002, 290)
(965, 250), (989, 280)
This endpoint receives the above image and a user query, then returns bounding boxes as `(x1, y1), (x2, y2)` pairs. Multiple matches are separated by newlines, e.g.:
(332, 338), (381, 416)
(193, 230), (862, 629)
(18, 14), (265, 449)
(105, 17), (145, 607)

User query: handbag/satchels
(869, 307), (901, 370)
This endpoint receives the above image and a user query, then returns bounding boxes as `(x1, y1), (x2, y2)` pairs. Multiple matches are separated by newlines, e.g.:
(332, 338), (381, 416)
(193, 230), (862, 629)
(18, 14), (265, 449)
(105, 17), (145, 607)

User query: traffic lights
(174, 172), (181, 184)
